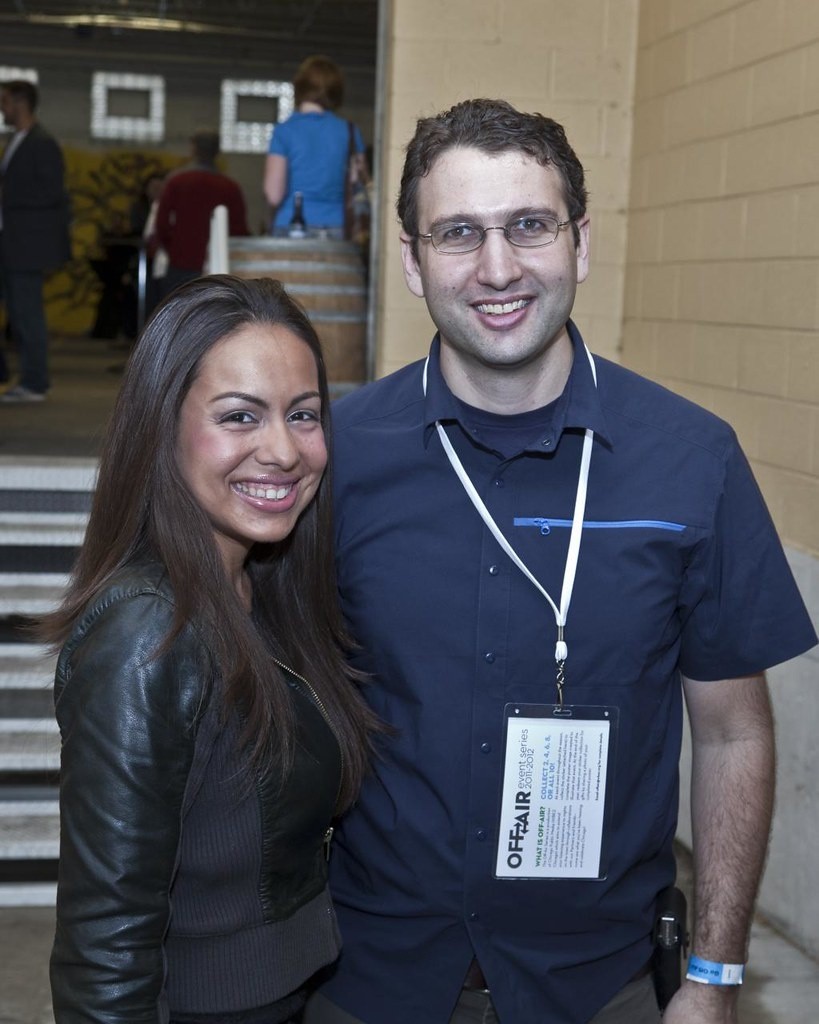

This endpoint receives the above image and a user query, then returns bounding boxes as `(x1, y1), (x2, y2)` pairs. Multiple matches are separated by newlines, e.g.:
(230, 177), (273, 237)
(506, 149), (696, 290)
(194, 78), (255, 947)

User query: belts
(461, 959), (491, 994)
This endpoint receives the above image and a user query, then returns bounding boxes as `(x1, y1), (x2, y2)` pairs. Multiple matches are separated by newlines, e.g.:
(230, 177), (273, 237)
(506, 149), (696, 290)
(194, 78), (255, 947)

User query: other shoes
(0, 385), (46, 403)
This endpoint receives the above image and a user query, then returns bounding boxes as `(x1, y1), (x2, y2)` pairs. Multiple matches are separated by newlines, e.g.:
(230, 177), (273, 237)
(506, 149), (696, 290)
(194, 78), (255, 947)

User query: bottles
(285, 192), (309, 239)
(345, 152), (370, 243)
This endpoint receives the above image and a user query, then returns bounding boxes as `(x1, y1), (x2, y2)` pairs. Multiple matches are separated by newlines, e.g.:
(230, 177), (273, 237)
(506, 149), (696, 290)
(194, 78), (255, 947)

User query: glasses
(411, 212), (576, 254)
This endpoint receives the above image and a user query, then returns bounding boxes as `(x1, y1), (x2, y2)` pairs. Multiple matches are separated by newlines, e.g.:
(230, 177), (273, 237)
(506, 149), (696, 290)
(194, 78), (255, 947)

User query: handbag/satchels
(346, 121), (370, 244)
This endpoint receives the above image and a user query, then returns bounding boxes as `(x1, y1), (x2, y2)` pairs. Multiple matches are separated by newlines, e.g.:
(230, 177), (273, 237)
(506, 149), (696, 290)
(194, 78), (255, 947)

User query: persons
(45, 273), (381, 1023)
(264, 57), (366, 241)
(0, 80), (68, 403)
(132, 175), (159, 234)
(329, 98), (819, 1024)
(153, 126), (255, 286)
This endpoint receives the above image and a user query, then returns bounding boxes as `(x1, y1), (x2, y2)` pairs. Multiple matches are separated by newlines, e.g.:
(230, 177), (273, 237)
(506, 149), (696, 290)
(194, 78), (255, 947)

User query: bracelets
(685, 956), (746, 985)
